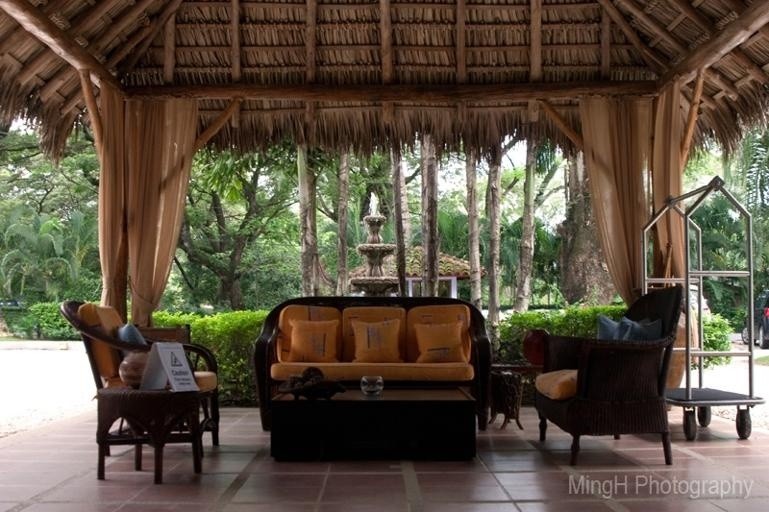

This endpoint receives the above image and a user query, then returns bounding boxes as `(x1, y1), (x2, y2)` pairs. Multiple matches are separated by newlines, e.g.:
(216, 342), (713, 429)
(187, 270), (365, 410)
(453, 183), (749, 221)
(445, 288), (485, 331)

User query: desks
(488, 359), (545, 431)
(94, 387), (202, 485)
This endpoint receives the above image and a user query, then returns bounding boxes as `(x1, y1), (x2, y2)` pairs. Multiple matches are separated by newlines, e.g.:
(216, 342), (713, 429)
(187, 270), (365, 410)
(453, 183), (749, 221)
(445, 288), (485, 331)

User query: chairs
(55, 296), (224, 485)
(532, 281), (688, 467)
(131, 322), (192, 346)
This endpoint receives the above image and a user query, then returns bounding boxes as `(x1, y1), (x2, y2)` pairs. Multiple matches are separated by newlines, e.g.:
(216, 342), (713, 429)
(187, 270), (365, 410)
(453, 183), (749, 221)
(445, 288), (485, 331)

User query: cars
(1, 297), (18, 306)
(688, 283), (712, 318)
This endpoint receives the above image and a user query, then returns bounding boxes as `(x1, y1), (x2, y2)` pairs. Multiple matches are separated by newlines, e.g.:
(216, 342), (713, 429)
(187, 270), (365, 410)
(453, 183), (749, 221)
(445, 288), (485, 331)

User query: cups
(361, 375), (383, 396)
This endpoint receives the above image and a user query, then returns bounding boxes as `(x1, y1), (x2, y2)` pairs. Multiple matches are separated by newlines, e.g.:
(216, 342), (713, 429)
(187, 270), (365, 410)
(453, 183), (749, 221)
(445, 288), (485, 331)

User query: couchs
(253, 293), (494, 433)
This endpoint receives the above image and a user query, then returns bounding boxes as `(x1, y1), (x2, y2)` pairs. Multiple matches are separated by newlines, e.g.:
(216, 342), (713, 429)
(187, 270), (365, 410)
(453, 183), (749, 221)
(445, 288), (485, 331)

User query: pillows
(412, 318), (469, 364)
(117, 318), (149, 348)
(594, 311), (664, 341)
(349, 315), (405, 364)
(287, 317), (343, 364)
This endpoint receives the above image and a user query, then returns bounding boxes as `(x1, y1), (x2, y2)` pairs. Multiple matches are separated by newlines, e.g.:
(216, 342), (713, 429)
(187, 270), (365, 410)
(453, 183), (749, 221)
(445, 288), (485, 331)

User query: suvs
(741, 289), (769, 350)
